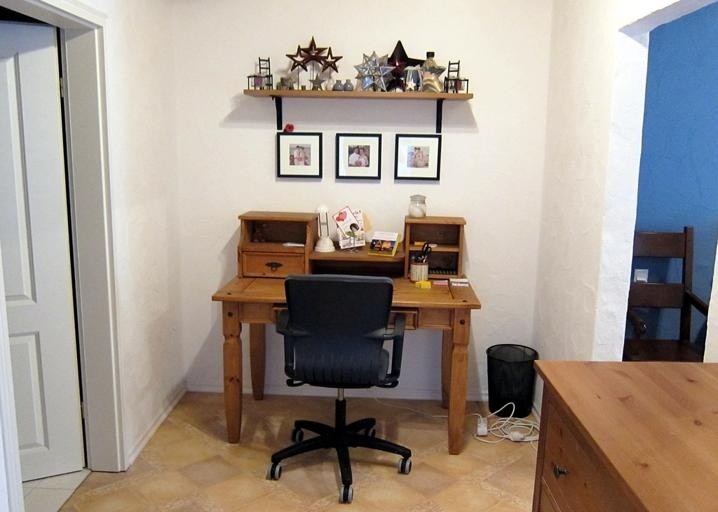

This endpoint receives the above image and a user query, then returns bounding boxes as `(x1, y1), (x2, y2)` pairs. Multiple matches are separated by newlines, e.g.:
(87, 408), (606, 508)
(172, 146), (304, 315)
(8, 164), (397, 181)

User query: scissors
(422, 243), (431, 257)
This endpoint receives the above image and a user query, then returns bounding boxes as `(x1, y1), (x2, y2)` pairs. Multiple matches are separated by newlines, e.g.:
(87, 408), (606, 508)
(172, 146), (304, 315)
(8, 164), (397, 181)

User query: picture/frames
(276, 132), (442, 180)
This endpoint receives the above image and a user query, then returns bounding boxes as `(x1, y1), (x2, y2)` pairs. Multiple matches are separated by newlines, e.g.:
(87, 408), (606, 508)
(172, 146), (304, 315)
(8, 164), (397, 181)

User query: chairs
(266, 274), (413, 503)
(622, 227), (710, 364)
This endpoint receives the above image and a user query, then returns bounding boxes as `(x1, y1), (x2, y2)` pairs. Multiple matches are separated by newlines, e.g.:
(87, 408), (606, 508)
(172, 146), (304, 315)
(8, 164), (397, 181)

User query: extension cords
(476, 416), (488, 436)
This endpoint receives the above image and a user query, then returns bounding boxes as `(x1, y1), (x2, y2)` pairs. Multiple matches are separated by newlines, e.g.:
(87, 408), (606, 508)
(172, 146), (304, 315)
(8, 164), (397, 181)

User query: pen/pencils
(414, 254), (428, 264)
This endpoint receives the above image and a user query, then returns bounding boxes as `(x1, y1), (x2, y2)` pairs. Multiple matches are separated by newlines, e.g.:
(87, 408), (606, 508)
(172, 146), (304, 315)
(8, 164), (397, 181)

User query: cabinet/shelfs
(533, 360), (718, 510)
(236, 211), (467, 280)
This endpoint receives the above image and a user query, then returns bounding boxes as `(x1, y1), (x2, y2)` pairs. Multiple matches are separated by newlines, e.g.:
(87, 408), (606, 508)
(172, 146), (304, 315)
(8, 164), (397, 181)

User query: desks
(211, 274), (482, 454)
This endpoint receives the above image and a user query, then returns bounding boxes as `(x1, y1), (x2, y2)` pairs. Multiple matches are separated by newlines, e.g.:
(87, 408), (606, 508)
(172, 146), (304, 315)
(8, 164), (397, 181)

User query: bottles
(408, 195), (427, 218)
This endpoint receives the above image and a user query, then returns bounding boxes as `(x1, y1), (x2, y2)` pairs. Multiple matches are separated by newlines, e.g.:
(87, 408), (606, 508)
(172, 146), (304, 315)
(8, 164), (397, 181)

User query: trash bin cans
(486, 344), (538, 418)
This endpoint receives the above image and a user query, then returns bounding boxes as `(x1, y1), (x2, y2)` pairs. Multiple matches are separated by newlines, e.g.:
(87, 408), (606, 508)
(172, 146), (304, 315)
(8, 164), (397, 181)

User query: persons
(407, 147), (422, 166)
(357, 148), (369, 167)
(299, 147), (306, 166)
(349, 146), (360, 166)
(293, 146), (299, 165)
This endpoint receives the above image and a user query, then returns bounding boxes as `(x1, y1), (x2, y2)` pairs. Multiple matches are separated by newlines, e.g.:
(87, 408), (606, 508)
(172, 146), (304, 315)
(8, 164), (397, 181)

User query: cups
(409, 263), (429, 283)
